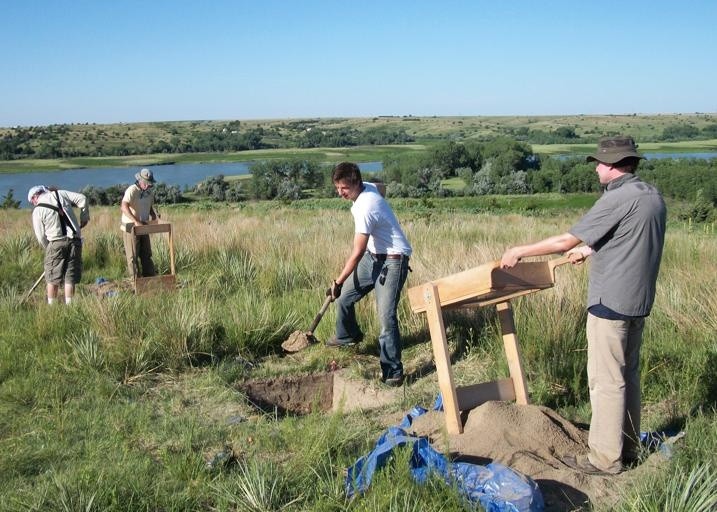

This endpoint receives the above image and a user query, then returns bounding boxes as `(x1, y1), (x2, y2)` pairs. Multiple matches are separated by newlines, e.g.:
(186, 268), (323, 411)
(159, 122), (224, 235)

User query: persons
(500, 138), (666, 474)
(27, 185), (90, 304)
(326, 162), (412, 387)
(119, 168), (160, 276)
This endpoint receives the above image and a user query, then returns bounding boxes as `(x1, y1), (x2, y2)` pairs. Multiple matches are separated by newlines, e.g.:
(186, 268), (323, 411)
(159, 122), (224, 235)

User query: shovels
(281, 283), (343, 351)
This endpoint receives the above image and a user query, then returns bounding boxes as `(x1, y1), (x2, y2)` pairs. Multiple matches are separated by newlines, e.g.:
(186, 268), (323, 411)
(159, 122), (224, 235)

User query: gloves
(327, 279), (342, 302)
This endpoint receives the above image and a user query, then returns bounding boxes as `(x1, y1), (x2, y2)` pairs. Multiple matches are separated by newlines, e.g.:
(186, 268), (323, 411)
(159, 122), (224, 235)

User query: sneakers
(385, 376), (402, 386)
(562, 453), (603, 473)
(328, 336), (363, 345)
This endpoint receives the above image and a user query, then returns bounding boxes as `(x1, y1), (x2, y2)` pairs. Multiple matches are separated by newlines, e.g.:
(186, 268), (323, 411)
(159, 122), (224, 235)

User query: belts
(371, 253), (401, 259)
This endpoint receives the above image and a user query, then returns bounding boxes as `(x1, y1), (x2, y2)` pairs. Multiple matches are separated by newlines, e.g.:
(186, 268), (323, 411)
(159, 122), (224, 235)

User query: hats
(586, 136), (647, 164)
(135, 168), (156, 184)
